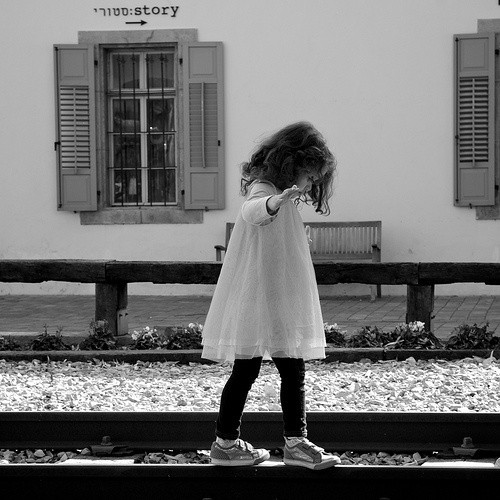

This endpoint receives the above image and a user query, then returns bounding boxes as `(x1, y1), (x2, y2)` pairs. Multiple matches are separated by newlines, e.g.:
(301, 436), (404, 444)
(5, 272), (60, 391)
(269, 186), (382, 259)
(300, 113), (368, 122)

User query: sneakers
(284, 436), (341, 470)
(210, 439), (270, 466)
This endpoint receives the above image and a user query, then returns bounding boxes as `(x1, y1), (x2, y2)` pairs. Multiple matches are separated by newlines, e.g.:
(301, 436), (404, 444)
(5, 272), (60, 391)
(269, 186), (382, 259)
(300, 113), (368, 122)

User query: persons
(200, 121), (342, 470)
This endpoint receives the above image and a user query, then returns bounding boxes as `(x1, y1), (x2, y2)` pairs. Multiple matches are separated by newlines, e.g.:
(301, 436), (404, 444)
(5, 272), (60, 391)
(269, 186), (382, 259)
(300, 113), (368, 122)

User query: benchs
(215, 221), (382, 298)
